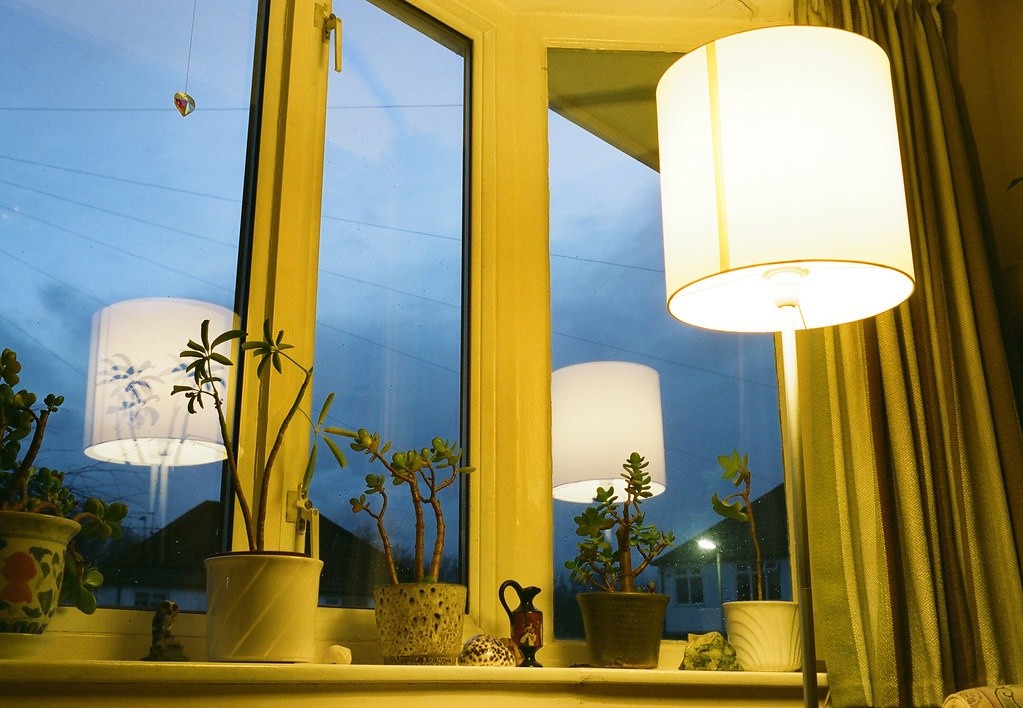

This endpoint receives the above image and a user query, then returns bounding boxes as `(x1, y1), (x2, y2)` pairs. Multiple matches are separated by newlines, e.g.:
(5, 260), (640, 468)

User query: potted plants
(564, 452), (674, 668)
(351, 430), (477, 665)
(0, 348), (126, 635)
(172, 321), (373, 661)
(711, 448), (804, 672)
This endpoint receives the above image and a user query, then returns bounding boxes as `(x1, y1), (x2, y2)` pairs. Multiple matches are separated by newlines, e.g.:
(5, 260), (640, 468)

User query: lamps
(550, 360), (668, 504)
(656, 24), (916, 334)
(83, 298), (239, 467)
